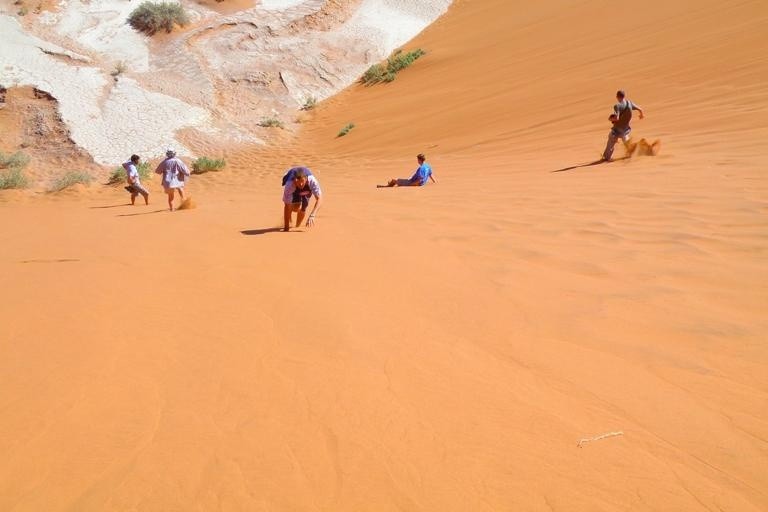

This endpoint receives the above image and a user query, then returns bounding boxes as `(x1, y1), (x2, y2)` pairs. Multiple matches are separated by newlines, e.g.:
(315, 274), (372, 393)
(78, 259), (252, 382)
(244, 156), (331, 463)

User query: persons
(122, 154), (150, 205)
(281, 167), (322, 232)
(600, 91), (644, 162)
(376, 153), (435, 188)
(155, 148), (190, 211)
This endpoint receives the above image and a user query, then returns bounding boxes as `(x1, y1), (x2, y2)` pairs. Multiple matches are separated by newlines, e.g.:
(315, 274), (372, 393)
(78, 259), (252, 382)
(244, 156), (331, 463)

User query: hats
(165, 147), (177, 158)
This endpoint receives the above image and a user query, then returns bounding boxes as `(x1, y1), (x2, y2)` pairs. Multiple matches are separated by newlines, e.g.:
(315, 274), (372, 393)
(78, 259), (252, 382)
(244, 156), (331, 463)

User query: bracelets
(309, 214), (314, 217)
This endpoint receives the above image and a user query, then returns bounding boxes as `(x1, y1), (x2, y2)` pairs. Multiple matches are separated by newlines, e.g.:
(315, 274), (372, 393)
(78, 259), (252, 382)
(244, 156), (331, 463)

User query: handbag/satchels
(619, 107), (631, 128)
